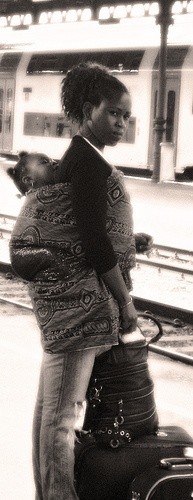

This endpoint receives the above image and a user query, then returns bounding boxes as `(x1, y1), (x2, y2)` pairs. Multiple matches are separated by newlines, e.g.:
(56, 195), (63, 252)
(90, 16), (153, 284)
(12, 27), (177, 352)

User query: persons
(5, 150), (84, 281)
(25, 60), (154, 500)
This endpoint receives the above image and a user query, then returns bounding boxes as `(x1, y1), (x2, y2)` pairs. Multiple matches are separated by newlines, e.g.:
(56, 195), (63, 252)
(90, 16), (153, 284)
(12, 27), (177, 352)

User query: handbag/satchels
(88, 310), (165, 451)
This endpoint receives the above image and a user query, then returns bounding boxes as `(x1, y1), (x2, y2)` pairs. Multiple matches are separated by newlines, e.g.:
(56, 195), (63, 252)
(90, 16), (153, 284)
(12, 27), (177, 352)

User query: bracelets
(119, 296), (133, 309)
